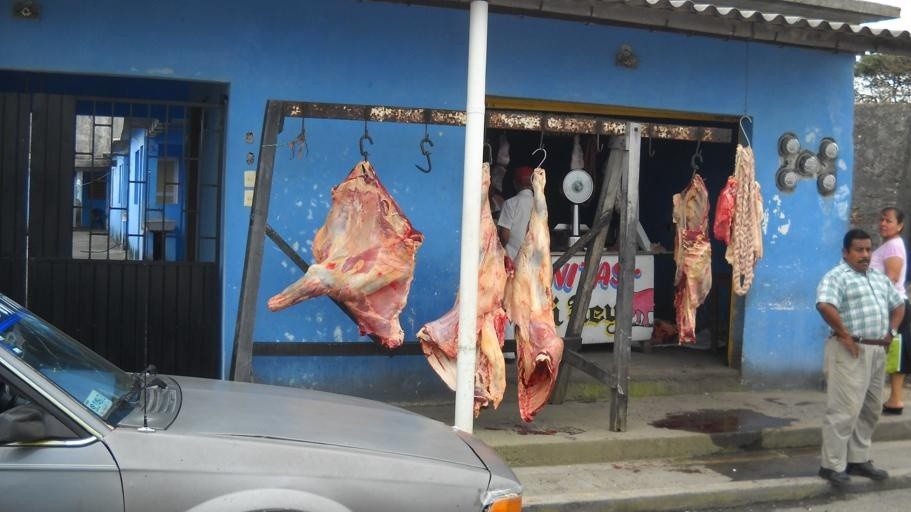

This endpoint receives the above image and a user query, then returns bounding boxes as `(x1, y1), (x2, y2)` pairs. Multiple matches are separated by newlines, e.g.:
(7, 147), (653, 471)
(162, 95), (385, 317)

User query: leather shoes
(848, 459), (888, 481)
(818, 465), (850, 490)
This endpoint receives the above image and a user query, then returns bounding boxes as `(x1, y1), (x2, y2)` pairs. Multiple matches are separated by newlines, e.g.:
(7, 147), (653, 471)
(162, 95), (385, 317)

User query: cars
(0, 293), (522, 511)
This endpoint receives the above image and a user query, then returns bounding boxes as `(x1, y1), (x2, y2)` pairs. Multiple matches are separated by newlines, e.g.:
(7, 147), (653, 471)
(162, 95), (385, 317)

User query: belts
(833, 331), (892, 346)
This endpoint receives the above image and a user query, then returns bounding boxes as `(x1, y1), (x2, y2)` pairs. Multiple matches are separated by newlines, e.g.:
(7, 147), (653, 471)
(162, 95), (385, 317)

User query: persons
(868, 204), (910, 416)
(815, 227), (909, 491)
(493, 163), (537, 269)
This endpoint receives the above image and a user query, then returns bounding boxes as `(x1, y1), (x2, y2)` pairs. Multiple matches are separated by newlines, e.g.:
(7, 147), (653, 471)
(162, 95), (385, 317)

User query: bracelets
(889, 327), (898, 337)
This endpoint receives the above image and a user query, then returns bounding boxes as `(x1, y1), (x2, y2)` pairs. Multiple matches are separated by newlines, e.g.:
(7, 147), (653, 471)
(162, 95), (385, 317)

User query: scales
(552, 168), (593, 250)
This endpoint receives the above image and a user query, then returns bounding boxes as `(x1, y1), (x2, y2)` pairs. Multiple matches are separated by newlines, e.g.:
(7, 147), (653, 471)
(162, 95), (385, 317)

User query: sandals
(882, 403), (904, 415)
